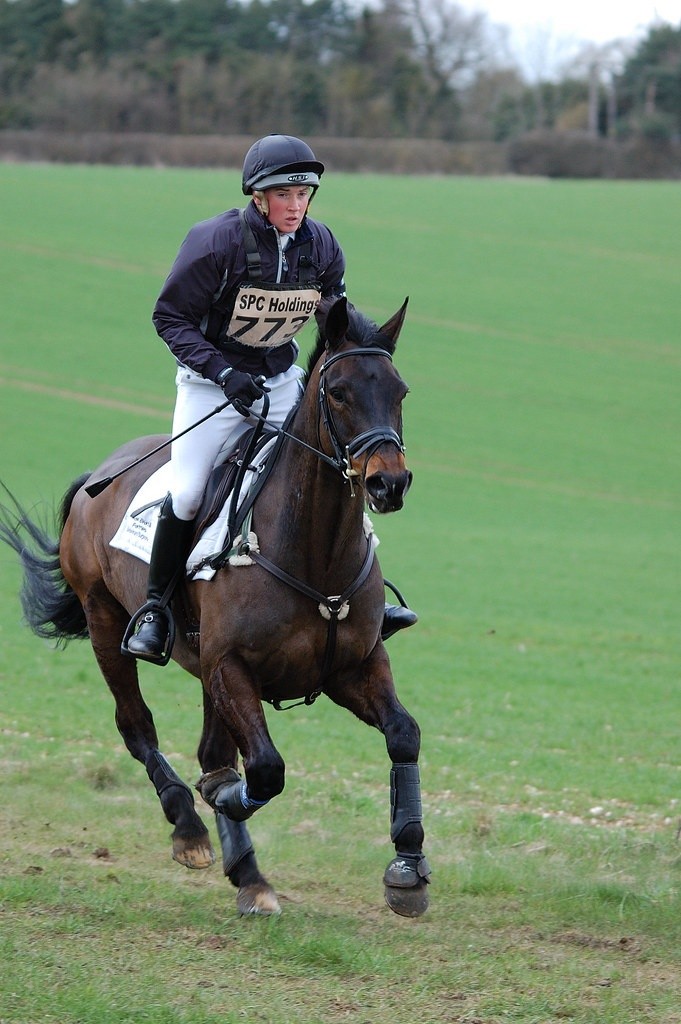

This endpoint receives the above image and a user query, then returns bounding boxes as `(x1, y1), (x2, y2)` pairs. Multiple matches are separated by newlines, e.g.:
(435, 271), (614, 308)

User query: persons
(127, 133), (418, 659)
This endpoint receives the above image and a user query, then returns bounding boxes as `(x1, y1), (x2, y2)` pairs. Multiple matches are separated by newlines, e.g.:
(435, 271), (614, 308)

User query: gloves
(218, 366), (272, 417)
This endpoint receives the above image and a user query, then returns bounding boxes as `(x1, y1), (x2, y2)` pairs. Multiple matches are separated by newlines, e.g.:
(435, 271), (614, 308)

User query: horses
(0, 294), (435, 921)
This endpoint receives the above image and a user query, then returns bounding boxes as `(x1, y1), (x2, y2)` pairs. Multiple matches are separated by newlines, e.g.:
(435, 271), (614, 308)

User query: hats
(251, 170), (320, 193)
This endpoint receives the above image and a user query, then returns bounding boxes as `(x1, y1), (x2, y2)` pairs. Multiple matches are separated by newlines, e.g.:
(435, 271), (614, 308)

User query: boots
(129, 492), (199, 658)
(381, 601), (419, 640)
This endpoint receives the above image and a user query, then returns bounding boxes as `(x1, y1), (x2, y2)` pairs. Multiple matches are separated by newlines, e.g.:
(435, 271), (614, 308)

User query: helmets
(242, 132), (325, 195)
